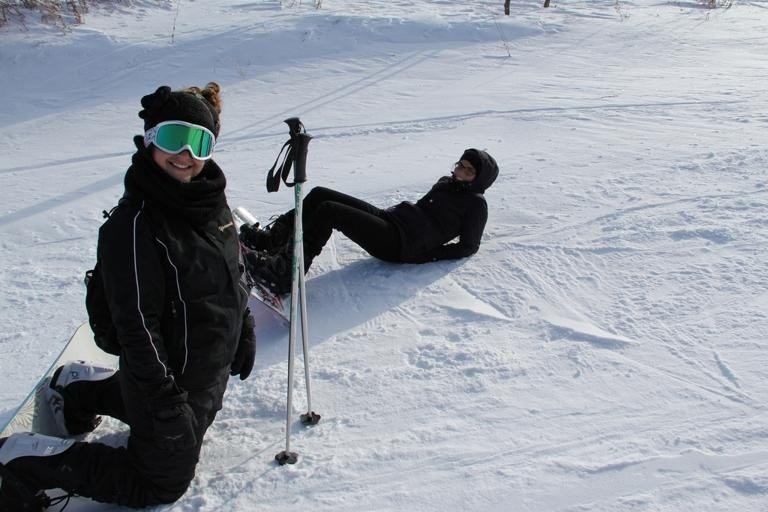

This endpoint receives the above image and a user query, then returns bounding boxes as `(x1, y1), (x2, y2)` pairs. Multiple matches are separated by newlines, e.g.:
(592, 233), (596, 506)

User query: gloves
(152, 395), (203, 472)
(230, 311), (258, 381)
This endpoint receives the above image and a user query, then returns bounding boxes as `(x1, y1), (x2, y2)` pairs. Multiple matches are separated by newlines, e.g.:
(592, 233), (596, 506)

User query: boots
(240, 209), (294, 256)
(244, 234), (312, 300)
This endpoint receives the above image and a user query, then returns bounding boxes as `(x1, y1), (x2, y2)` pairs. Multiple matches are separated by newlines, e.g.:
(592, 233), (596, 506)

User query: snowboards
(232, 207), (291, 324)
(0, 320), (120, 512)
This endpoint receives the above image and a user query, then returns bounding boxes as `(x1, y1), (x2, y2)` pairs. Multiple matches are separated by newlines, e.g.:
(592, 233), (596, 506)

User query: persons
(237, 145), (500, 299)
(0, 78), (256, 512)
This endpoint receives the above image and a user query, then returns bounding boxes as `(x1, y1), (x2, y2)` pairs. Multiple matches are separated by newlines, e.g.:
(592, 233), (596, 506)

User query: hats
(460, 148), (499, 194)
(137, 85), (220, 140)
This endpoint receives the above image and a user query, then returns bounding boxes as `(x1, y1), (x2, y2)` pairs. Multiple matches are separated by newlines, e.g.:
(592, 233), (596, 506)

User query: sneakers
(0, 435), (52, 512)
(46, 364), (95, 437)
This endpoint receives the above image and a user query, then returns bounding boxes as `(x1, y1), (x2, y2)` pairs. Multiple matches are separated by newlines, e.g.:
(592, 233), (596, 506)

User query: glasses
(144, 119), (217, 161)
(455, 161), (478, 177)
